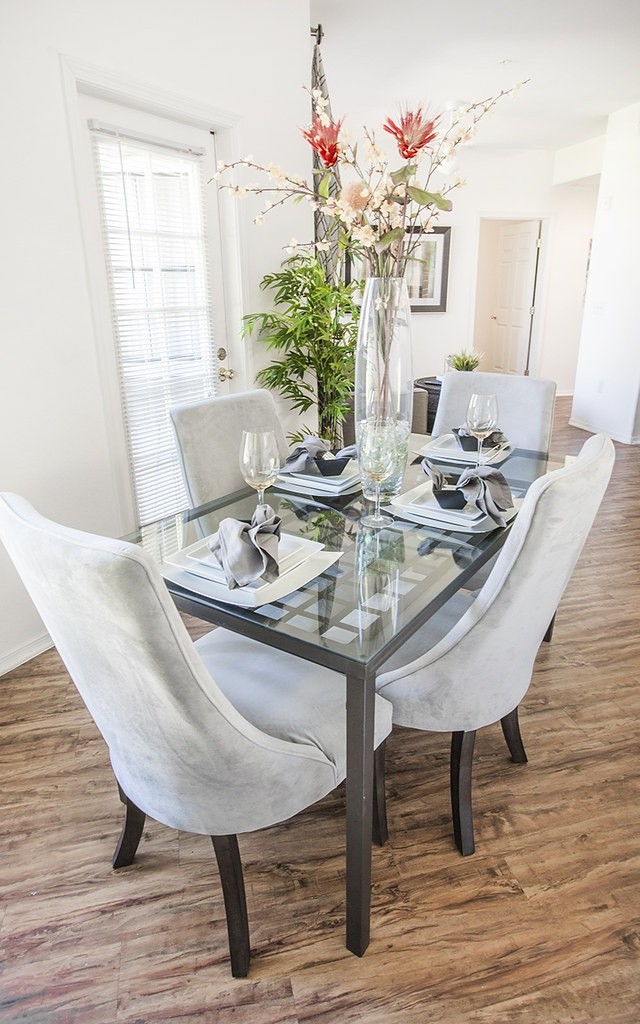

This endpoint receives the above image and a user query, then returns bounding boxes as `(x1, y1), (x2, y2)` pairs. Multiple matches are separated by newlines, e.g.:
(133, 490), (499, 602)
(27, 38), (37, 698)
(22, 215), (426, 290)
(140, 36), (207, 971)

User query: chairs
(168, 389), (293, 540)
(431, 372), (555, 490)
(0, 490), (396, 981)
(374, 433), (616, 858)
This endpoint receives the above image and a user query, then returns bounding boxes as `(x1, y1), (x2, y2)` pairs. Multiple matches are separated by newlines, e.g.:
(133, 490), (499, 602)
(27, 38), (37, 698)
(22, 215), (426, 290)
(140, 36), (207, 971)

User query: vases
(356, 274), (412, 501)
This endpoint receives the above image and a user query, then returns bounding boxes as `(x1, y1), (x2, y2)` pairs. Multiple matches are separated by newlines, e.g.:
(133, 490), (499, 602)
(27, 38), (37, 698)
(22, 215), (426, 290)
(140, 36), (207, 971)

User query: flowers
(203, 73), (542, 419)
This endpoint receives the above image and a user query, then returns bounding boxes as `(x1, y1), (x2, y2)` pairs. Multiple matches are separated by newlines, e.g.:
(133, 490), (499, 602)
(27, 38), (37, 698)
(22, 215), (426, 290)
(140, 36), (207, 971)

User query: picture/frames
(343, 222), (454, 315)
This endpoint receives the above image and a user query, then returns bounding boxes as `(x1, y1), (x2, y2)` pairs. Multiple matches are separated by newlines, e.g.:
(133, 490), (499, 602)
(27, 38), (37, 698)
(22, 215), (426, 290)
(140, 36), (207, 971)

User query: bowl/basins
(432, 477), (468, 510)
(451, 429), (478, 452)
(313, 449), (352, 476)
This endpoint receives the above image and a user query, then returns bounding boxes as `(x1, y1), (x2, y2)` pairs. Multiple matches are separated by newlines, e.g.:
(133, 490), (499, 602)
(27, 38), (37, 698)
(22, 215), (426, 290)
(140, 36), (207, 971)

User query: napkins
(206, 504), (281, 590)
(452, 425), (510, 452)
(421, 457), (511, 529)
(279, 430), (358, 472)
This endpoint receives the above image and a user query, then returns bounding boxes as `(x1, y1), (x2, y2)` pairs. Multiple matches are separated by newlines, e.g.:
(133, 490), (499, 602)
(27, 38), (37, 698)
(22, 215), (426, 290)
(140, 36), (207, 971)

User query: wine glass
(466, 392), (497, 469)
(238, 428), (280, 505)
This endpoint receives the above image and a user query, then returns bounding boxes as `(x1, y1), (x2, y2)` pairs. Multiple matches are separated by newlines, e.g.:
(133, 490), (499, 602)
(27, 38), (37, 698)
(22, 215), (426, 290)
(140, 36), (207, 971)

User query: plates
(160, 532), (344, 607)
(271, 460), (363, 496)
(380, 479), (525, 533)
(412, 434), (519, 465)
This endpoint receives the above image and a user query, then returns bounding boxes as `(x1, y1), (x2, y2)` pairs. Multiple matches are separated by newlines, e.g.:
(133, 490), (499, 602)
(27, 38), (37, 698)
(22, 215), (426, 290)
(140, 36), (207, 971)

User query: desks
(112, 436), (568, 956)
